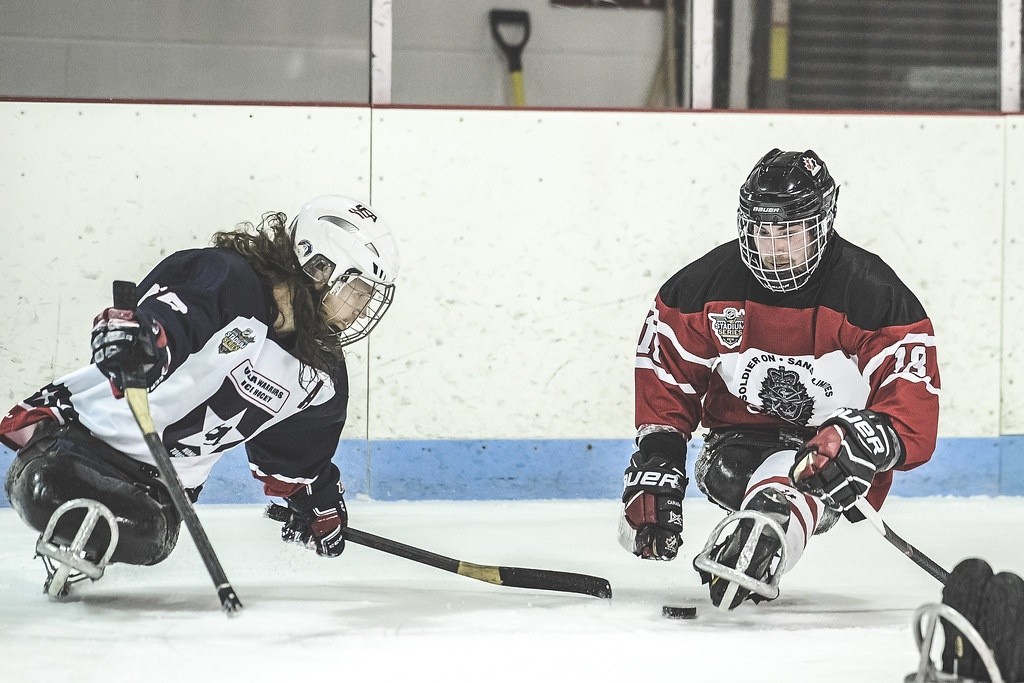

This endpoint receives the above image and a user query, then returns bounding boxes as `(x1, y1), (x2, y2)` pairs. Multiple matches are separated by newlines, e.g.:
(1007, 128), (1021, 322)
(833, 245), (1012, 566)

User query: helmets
(286, 194), (400, 352)
(737, 148), (841, 293)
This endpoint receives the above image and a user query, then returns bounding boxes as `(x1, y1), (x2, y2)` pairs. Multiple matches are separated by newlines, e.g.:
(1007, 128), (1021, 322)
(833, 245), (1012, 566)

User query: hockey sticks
(857, 493), (953, 587)
(265, 501), (614, 602)
(116, 275), (243, 622)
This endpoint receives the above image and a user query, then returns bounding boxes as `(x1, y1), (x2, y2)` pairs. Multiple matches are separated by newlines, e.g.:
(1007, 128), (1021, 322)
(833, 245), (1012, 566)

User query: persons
(620, 148), (940, 611)
(910, 559), (1024, 683)
(0, 198), (401, 602)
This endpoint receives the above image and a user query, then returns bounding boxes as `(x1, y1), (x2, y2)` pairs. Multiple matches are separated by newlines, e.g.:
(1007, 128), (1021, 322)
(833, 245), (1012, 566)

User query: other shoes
(940, 559), (1024, 683)
(710, 488), (791, 610)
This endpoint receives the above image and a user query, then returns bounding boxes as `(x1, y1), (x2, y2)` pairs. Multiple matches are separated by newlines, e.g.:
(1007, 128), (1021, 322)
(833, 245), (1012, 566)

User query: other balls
(663, 598), (698, 622)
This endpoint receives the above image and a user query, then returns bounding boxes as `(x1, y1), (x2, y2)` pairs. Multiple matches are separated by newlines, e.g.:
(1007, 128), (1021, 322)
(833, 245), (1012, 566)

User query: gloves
(90, 307), (171, 399)
(788, 407), (895, 513)
(281, 462), (348, 558)
(617, 451), (689, 561)
